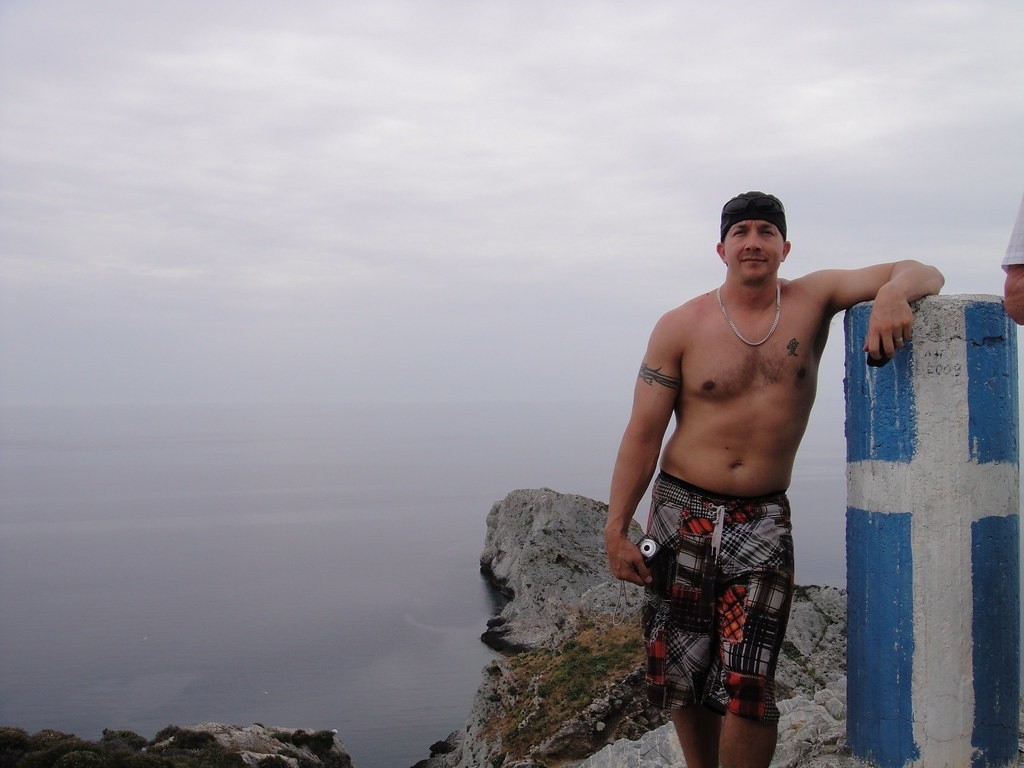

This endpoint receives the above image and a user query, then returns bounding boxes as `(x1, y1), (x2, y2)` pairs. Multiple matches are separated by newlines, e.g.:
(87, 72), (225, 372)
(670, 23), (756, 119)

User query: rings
(894, 337), (904, 343)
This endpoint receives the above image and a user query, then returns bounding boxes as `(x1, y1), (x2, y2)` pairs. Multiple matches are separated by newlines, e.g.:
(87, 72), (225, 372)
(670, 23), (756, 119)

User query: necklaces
(717, 280), (780, 346)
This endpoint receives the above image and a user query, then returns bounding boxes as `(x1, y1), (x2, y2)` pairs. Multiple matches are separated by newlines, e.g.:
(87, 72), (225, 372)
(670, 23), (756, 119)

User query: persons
(1001, 197), (1024, 329)
(606, 190), (945, 767)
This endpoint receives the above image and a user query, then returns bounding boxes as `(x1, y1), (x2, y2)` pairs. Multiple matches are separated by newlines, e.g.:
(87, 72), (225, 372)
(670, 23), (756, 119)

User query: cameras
(636, 534), (663, 568)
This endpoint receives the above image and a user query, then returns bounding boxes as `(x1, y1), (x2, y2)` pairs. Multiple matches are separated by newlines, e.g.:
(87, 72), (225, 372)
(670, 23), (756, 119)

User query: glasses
(721, 197), (786, 216)
